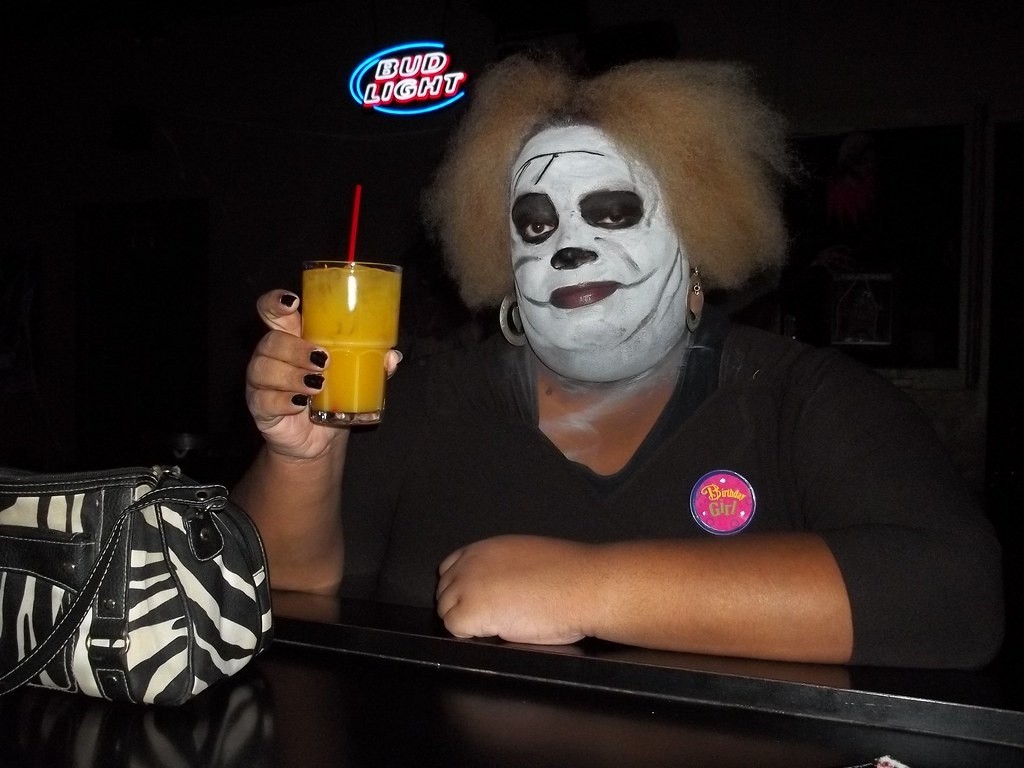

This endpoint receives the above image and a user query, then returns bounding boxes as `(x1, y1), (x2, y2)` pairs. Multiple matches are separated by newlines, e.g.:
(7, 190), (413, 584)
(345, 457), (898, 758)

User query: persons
(231, 49), (1006, 672)
(392, 239), (491, 369)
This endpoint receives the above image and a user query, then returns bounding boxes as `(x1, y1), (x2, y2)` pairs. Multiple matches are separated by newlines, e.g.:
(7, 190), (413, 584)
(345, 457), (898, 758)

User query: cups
(301, 264), (402, 426)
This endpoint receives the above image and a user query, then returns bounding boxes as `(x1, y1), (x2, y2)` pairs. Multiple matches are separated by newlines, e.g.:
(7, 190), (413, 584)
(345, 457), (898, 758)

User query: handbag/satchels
(0, 466), (273, 708)
